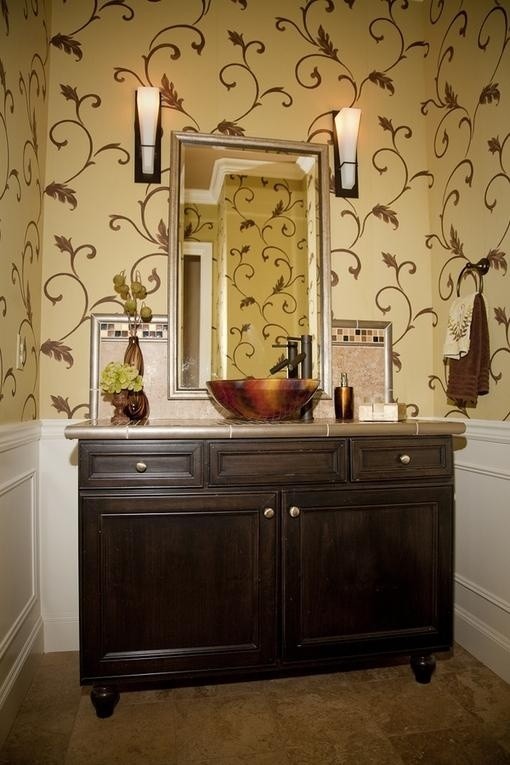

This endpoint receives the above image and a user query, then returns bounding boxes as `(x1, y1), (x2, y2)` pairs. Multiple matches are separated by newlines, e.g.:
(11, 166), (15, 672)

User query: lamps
(332, 109), (363, 198)
(131, 85), (161, 182)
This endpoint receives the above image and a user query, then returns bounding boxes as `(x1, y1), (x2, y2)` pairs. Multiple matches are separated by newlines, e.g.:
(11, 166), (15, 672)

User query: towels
(443, 292), (489, 410)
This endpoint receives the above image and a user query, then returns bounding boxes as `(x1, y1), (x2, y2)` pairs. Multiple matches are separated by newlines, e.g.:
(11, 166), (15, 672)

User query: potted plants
(113, 270), (152, 425)
(100, 361), (144, 425)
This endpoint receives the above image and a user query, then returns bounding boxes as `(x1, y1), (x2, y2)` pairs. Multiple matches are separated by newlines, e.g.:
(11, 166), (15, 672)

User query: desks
(65, 417), (467, 720)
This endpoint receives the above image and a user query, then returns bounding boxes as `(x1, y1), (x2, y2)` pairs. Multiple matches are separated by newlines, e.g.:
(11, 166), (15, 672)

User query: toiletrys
(334, 373), (354, 420)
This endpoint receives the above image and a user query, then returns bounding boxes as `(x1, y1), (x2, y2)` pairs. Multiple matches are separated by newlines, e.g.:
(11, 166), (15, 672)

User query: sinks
(205, 379), (321, 420)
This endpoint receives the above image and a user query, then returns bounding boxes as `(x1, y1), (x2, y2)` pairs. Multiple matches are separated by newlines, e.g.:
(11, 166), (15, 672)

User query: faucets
(270, 341), (299, 378)
(286, 335), (314, 421)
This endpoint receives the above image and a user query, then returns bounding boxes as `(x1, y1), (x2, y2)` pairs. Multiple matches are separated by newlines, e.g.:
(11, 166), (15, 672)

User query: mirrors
(167, 128), (333, 399)
